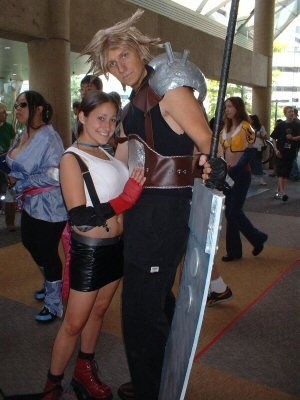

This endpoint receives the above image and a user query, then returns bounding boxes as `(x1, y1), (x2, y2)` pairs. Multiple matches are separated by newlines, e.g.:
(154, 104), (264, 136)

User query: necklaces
(74, 141), (99, 148)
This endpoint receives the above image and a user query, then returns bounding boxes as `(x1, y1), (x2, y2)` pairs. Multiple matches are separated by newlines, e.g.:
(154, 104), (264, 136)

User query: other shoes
(117, 383), (136, 400)
(42, 379), (65, 400)
(260, 180), (267, 185)
(73, 357), (111, 400)
(251, 233), (268, 256)
(282, 195), (288, 201)
(34, 308), (57, 323)
(222, 251), (243, 261)
(35, 286), (47, 302)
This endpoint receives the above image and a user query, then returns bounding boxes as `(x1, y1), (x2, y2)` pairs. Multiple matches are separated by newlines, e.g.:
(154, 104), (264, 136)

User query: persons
(79, 9), (228, 400)
(216, 97), (268, 262)
(208, 106), (300, 200)
(0, 75), (124, 232)
(6, 91), (68, 322)
(42, 91), (147, 400)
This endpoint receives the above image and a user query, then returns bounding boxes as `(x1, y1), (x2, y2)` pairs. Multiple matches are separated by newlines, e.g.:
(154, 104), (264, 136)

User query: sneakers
(206, 287), (233, 307)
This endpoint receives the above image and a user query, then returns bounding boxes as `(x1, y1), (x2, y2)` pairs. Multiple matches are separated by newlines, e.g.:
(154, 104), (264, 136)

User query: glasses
(14, 102), (29, 109)
